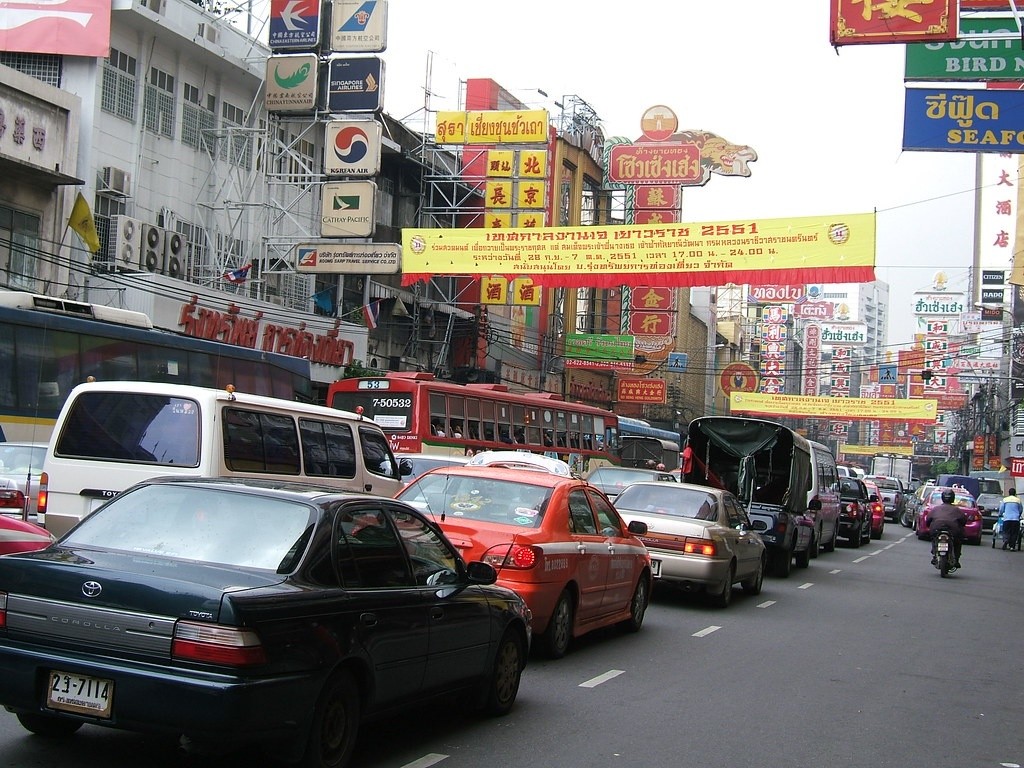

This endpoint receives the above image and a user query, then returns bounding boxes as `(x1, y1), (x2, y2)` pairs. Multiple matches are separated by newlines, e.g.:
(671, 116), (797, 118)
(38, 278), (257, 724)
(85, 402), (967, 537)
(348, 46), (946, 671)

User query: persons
(432, 419), (445, 437)
(926, 489), (968, 568)
(451, 423), (463, 438)
(999, 488), (1023, 551)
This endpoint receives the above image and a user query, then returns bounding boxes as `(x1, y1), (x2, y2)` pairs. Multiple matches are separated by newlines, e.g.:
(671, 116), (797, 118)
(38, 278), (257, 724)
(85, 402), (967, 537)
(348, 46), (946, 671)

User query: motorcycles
(928, 525), (966, 579)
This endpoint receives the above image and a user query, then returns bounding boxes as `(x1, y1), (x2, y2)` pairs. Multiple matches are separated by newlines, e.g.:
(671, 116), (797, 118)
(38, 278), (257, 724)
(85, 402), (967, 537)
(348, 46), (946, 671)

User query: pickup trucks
(680, 417), (822, 579)
(838, 475), (878, 548)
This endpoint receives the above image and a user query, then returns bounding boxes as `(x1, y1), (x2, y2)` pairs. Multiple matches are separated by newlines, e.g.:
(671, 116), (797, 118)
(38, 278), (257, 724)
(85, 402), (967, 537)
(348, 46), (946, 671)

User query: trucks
(871, 453), (913, 494)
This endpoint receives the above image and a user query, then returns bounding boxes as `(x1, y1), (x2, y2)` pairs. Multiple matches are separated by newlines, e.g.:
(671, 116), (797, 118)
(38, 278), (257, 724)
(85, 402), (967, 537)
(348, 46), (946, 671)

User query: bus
(0, 289), (311, 442)
(325, 374), (621, 479)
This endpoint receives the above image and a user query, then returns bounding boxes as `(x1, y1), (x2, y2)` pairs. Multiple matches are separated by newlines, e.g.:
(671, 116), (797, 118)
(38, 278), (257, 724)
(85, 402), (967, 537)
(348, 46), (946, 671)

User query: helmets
(941, 489), (956, 503)
(655, 463), (666, 471)
(646, 460), (656, 469)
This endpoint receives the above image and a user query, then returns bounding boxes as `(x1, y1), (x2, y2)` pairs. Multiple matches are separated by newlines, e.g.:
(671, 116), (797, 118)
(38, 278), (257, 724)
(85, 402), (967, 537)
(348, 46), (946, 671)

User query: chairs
(430, 424), (592, 451)
(37, 381), (61, 411)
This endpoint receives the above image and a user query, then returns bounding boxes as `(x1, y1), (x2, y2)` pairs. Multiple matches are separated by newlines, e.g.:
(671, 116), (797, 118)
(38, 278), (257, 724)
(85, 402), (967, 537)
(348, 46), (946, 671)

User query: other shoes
(1009, 547), (1016, 551)
(1001, 540), (1008, 550)
(950, 560), (962, 568)
(931, 555), (938, 565)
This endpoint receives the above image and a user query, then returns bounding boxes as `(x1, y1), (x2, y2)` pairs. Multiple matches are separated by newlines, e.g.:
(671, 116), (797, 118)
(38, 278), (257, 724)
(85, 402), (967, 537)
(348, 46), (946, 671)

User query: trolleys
(993, 514), (1024, 551)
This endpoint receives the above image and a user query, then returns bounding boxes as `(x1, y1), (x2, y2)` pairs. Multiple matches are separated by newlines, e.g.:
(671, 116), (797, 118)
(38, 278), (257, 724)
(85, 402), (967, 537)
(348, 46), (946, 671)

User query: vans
(36, 377), (413, 542)
(804, 438), (850, 560)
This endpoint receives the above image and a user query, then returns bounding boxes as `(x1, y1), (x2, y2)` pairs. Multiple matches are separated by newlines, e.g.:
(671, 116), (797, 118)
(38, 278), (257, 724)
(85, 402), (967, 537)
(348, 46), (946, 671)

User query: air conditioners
(101, 166), (131, 196)
(107, 215), (188, 281)
(195, 22), (223, 45)
(141, 0), (167, 15)
(368, 354), (382, 368)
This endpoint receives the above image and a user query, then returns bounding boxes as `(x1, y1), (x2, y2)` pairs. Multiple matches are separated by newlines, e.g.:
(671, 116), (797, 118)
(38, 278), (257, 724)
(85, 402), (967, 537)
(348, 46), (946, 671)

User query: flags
(223, 264), (252, 283)
(311, 285), (341, 312)
(68, 192), (101, 253)
(423, 303), (435, 337)
(363, 300), (380, 329)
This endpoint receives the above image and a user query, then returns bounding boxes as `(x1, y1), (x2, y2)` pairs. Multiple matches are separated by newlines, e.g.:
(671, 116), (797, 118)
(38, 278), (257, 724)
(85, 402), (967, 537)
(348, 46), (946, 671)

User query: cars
(1, 442), (50, 525)
(352, 451), (654, 665)
(389, 444), (686, 508)
(608, 482), (770, 607)
(0, 474), (534, 768)
(836, 465), (1007, 544)
